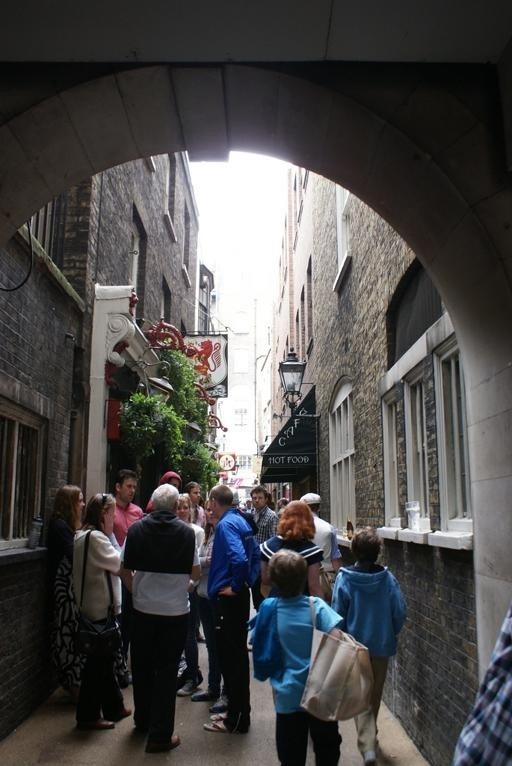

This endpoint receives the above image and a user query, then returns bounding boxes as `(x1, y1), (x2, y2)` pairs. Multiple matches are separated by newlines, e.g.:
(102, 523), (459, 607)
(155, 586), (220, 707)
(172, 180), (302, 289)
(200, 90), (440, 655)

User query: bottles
(28, 516), (44, 550)
(345, 515), (354, 541)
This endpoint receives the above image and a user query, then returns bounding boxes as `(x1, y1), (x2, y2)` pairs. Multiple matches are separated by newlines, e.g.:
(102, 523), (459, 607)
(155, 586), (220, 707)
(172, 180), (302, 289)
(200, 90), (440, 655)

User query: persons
(247, 487), (407, 765)
(39, 468), (258, 753)
(453, 603), (512, 766)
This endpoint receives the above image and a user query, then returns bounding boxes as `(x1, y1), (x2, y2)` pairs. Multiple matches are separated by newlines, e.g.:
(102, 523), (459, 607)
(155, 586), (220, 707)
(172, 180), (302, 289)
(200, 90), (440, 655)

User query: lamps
(278, 347), (321, 419)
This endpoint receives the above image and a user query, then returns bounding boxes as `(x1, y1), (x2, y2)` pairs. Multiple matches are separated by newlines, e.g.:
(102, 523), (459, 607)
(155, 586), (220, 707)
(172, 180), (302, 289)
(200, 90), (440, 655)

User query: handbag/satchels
(300, 627), (371, 723)
(70, 613), (121, 656)
(320, 567), (337, 606)
(249, 623), (280, 682)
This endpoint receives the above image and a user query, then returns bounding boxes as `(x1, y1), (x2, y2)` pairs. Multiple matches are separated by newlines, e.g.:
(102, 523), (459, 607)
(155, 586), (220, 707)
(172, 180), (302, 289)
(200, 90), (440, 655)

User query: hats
(300, 493), (322, 506)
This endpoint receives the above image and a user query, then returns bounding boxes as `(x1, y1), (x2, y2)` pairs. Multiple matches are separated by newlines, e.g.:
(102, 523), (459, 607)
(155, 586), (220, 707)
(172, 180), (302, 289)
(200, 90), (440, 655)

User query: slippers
(204, 713), (248, 734)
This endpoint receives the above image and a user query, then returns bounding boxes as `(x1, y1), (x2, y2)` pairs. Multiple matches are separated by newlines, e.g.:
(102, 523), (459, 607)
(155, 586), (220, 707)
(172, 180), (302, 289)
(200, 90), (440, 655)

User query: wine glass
(404, 500), (421, 533)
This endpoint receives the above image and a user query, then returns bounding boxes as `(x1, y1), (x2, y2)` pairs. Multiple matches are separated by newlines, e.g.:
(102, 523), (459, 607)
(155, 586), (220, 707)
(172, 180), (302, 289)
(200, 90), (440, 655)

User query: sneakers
(116, 665), (130, 689)
(208, 696), (233, 712)
(104, 704), (132, 722)
(177, 673), (199, 695)
(75, 718), (118, 729)
(134, 719), (153, 733)
(146, 731), (181, 753)
(191, 688), (218, 702)
(44, 694), (73, 709)
(364, 749), (377, 766)
